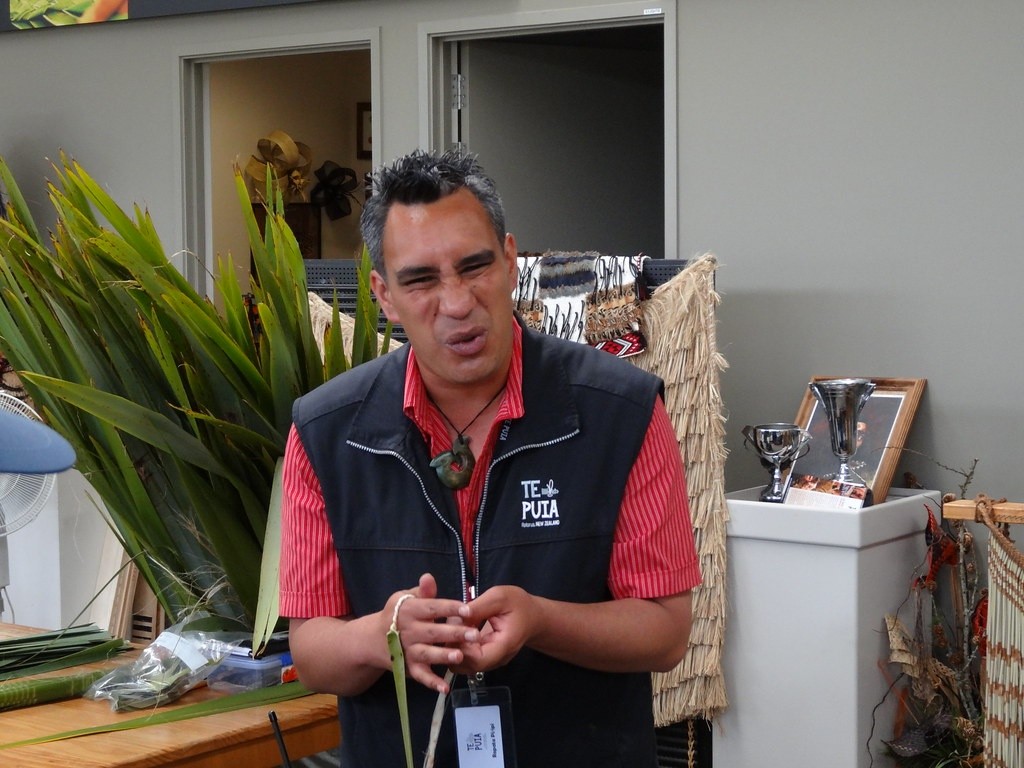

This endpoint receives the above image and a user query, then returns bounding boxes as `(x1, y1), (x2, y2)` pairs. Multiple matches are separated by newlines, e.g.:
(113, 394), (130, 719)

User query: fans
(0, 393), (56, 625)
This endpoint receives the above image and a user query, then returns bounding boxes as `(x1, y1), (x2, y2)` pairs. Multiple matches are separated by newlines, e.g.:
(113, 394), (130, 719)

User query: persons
(276, 145), (704, 767)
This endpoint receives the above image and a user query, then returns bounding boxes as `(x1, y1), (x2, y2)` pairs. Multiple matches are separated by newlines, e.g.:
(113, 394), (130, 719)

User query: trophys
(809, 376), (877, 486)
(743, 423), (813, 502)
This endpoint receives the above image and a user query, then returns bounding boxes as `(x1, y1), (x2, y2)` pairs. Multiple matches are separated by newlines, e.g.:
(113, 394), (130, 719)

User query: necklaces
(424, 379), (508, 492)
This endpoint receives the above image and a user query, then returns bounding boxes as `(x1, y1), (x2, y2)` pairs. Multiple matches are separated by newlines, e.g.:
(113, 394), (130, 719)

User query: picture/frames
(357, 100), (373, 161)
(250, 203), (322, 287)
(780, 376), (928, 506)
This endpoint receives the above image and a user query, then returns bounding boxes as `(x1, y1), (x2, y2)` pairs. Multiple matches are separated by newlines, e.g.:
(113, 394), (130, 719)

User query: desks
(0, 617), (341, 768)
(710, 484), (942, 768)
